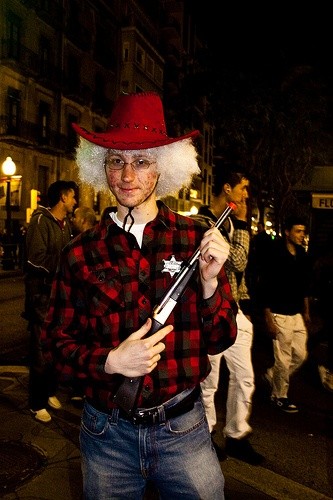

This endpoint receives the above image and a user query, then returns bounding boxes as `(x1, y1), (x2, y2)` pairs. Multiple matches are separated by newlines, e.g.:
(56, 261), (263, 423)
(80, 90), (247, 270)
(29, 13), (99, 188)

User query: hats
(72, 90), (199, 150)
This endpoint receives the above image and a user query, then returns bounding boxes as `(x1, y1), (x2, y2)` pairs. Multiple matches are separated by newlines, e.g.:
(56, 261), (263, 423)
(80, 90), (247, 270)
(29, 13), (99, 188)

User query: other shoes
(262, 375), (299, 414)
(211, 436), (265, 467)
(29, 408), (52, 422)
(48, 396), (62, 409)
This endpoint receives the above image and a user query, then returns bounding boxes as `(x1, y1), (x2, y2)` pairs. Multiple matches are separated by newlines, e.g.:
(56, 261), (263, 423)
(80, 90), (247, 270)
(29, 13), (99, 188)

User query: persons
(0, 228), (18, 268)
(40, 92), (237, 500)
(262, 217), (312, 411)
(308, 240), (333, 392)
(189, 169), (266, 465)
(21, 180), (77, 422)
(250, 221), (272, 253)
(70, 206), (98, 234)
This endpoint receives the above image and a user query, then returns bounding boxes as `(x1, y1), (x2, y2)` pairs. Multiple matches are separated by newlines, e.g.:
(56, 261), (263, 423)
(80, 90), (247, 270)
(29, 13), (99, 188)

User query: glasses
(105, 158), (157, 171)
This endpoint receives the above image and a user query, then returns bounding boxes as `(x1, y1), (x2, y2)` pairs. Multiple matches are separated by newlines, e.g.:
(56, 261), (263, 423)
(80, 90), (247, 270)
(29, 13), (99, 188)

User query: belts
(85, 384), (203, 427)
